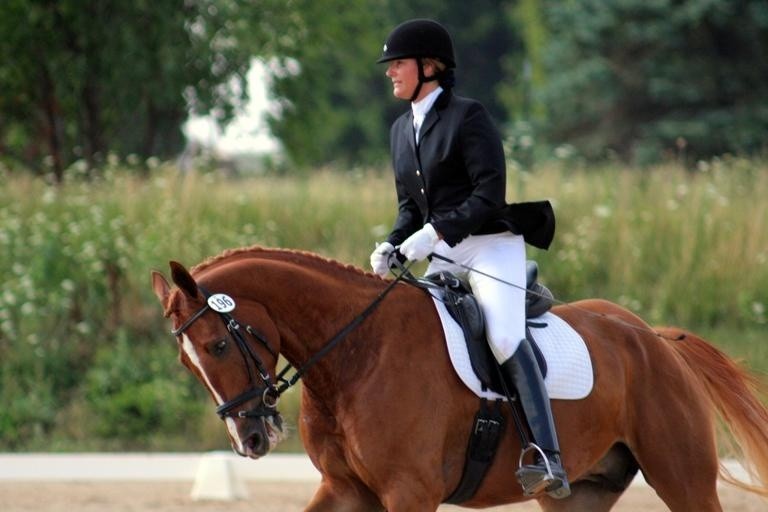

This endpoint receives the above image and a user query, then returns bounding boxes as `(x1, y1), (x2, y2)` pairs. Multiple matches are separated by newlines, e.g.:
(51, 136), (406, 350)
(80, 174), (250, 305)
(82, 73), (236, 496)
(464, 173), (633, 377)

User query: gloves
(370, 241), (396, 280)
(399, 223), (439, 263)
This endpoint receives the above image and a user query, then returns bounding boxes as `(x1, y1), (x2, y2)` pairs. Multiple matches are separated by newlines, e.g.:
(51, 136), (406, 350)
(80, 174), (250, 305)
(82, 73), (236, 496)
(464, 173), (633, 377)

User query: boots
(498, 338), (572, 502)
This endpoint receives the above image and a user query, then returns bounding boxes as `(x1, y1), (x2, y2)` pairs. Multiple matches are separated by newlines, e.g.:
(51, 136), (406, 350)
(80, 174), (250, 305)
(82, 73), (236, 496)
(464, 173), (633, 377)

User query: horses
(144, 243), (768, 512)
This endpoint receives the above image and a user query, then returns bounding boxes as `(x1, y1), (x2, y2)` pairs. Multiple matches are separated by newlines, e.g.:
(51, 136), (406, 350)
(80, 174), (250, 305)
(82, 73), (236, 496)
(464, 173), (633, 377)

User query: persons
(370, 18), (572, 500)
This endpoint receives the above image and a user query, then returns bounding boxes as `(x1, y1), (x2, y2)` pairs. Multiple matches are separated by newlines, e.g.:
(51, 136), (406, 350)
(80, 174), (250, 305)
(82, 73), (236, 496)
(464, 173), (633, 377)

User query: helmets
(374, 18), (458, 72)
